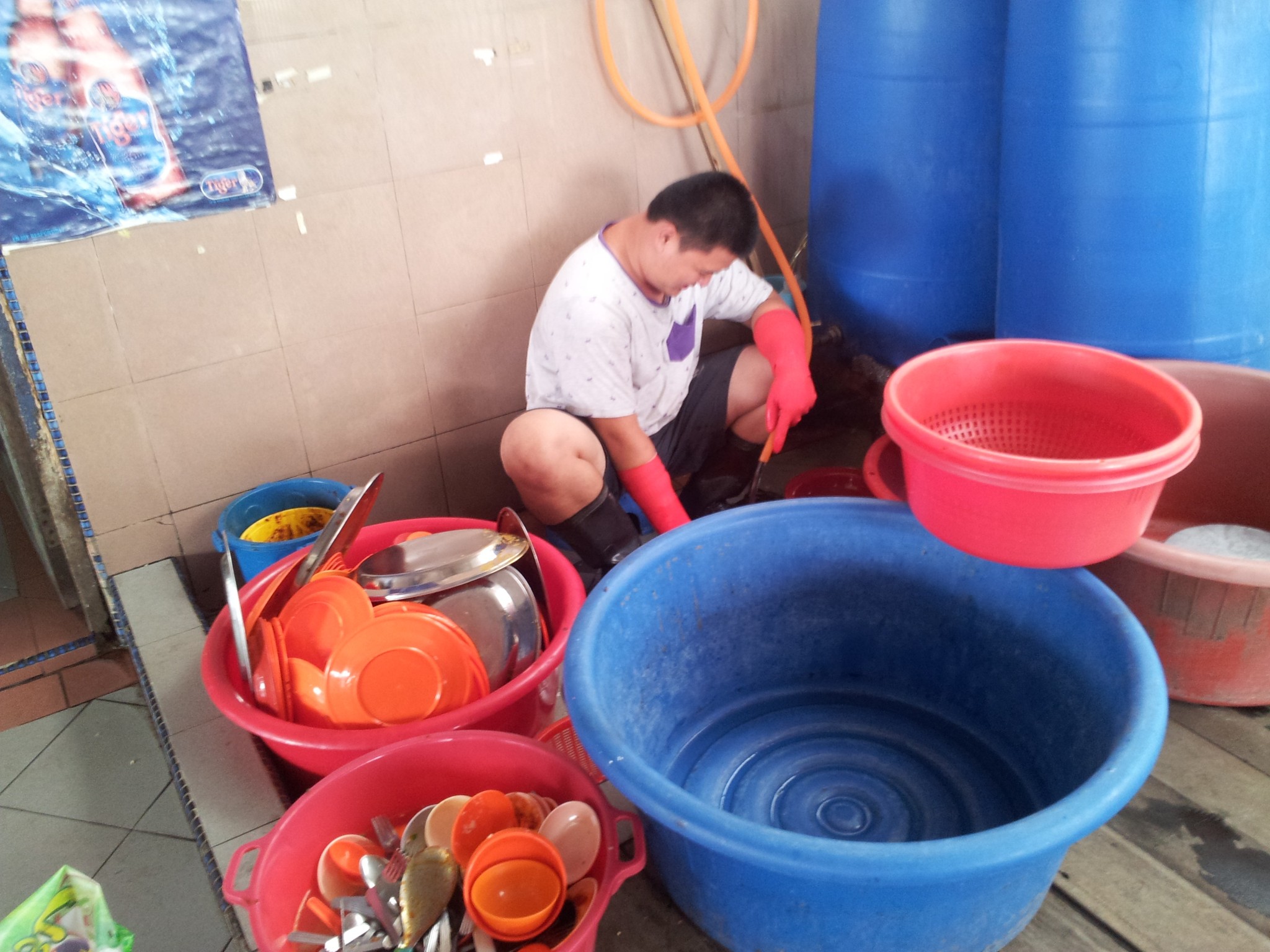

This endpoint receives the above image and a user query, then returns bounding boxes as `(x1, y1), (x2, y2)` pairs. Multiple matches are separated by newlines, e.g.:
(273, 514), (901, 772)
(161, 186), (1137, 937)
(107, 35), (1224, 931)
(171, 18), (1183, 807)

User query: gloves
(751, 307), (816, 454)
(617, 459), (691, 535)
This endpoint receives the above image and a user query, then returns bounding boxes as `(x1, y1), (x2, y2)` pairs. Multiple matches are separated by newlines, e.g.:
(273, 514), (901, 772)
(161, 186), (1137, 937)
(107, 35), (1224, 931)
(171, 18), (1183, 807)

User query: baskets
(883, 340), (1203, 477)
(535, 716), (608, 784)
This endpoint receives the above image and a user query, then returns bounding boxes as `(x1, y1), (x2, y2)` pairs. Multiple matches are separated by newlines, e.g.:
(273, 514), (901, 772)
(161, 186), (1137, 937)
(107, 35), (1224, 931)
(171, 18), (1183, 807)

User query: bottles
(7, 1), (82, 182)
(56, 9), (189, 214)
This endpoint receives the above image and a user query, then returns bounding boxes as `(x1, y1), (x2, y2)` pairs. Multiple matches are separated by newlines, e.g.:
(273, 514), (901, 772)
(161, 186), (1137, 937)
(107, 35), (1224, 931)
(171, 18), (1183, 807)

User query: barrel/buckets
(800, 1), (1006, 372)
(994, 1), (1270, 378)
(212, 476), (357, 583)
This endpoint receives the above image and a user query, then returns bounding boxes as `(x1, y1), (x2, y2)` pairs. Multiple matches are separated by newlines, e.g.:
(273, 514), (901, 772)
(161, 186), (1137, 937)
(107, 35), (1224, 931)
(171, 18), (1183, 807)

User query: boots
(547, 484), (641, 576)
(680, 427), (779, 519)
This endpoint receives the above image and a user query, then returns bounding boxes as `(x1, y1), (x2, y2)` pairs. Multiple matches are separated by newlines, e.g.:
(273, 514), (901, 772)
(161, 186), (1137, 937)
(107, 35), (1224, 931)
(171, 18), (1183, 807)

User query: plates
(219, 470), (553, 729)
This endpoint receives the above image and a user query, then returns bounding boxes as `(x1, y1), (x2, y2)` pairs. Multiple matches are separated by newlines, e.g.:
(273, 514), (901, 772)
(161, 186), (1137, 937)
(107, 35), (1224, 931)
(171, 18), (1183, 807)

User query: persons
(495, 173), (818, 584)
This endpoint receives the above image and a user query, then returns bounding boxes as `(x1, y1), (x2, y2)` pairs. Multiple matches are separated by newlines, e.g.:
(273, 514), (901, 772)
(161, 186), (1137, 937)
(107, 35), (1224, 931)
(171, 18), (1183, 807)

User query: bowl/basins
(861, 431), (904, 502)
(1082, 357), (1270, 707)
(878, 406), (1201, 571)
(782, 465), (874, 498)
(289, 785), (602, 952)
(223, 729), (643, 952)
(561, 492), (1172, 950)
(199, 513), (589, 777)
